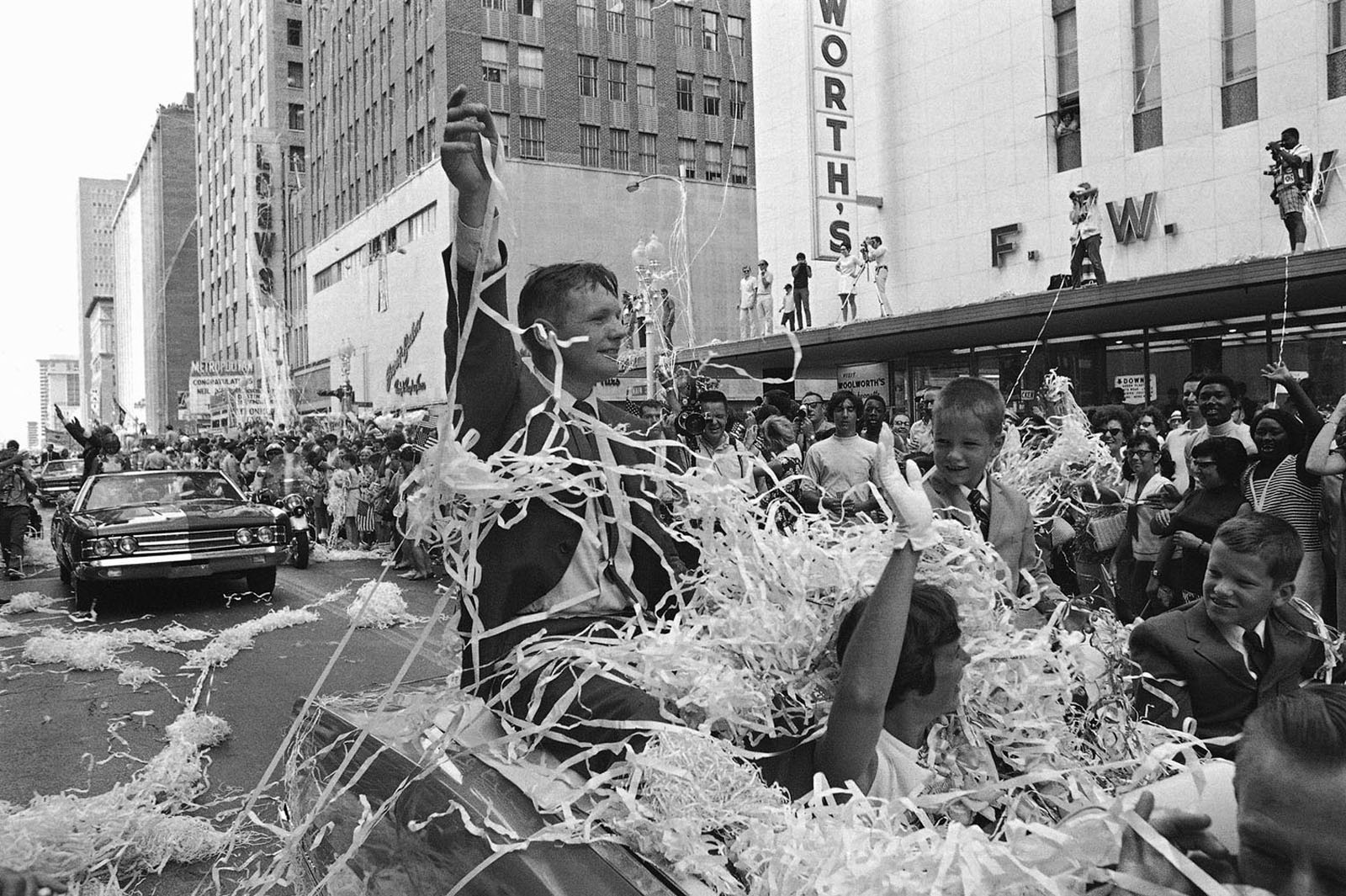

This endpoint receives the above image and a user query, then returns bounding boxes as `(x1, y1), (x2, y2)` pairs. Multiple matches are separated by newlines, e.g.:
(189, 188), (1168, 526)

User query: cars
(29, 464), (42, 480)
(54, 467), (294, 613)
(39, 457), (84, 502)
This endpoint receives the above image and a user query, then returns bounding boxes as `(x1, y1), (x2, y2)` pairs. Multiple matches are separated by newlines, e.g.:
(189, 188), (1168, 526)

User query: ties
(578, 399), (618, 583)
(1245, 627), (1269, 682)
(967, 488), (989, 543)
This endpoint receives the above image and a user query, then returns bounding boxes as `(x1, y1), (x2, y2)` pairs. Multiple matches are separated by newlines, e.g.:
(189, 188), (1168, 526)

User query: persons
(736, 264), (757, 340)
(778, 283), (796, 332)
(756, 259), (774, 337)
(1068, 182), (1107, 289)
(860, 236), (894, 318)
(620, 288), (647, 351)
(922, 376), (1068, 623)
(1053, 112), (1078, 139)
(835, 241), (865, 322)
(823, 420), (996, 833)
(1274, 127), (1312, 256)
(790, 252), (812, 331)
(659, 288), (677, 350)
(639, 358), (1346, 642)
(1131, 509), (1328, 758)
(1109, 682), (1345, 895)
(437, 84), (815, 809)
(1, 404), (438, 583)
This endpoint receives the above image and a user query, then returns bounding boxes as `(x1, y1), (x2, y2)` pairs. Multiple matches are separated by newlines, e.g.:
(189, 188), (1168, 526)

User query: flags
(355, 500), (376, 532)
(419, 414), (438, 429)
(414, 430), (438, 452)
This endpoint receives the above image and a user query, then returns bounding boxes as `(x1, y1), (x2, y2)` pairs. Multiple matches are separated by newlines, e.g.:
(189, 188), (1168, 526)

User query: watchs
(1150, 568), (1162, 578)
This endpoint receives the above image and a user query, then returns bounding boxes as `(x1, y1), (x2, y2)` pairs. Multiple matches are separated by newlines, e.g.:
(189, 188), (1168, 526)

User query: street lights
(632, 229), (664, 401)
(626, 173), (695, 344)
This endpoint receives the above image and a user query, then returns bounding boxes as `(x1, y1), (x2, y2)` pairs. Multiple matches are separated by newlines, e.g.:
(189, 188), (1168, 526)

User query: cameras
(1266, 140), (1286, 161)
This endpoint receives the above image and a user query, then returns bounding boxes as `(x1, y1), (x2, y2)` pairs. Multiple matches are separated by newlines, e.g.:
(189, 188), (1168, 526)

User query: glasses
(1096, 428), (1122, 436)
(758, 264), (765, 266)
(267, 452), (282, 456)
(1136, 421), (1154, 427)
(744, 270), (749, 273)
(914, 395), (924, 402)
(1127, 450), (1152, 458)
(805, 401), (823, 408)
(1193, 459), (1212, 469)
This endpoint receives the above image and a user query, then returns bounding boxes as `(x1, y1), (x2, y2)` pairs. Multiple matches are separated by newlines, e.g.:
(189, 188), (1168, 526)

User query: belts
(876, 266), (887, 271)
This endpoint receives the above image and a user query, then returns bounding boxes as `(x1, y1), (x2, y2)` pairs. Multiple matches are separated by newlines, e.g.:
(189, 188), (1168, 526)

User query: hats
(1167, 410), (1183, 423)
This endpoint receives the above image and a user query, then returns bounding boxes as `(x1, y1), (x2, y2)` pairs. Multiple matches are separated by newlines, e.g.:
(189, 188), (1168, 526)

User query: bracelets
(1196, 541), (1204, 549)
(1325, 420), (1339, 425)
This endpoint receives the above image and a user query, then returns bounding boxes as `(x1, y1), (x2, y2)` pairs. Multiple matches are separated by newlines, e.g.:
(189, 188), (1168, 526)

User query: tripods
(835, 259), (884, 322)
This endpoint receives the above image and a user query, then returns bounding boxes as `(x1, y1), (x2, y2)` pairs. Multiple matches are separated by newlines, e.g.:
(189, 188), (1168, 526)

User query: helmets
(264, 443), (283, 459)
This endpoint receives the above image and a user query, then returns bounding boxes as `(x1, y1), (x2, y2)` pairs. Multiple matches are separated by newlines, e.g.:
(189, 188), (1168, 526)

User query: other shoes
(340, 540), (436, 581)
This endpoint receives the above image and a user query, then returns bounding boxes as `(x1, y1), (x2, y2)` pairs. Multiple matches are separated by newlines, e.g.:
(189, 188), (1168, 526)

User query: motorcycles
(249, 452), (325, 569)
